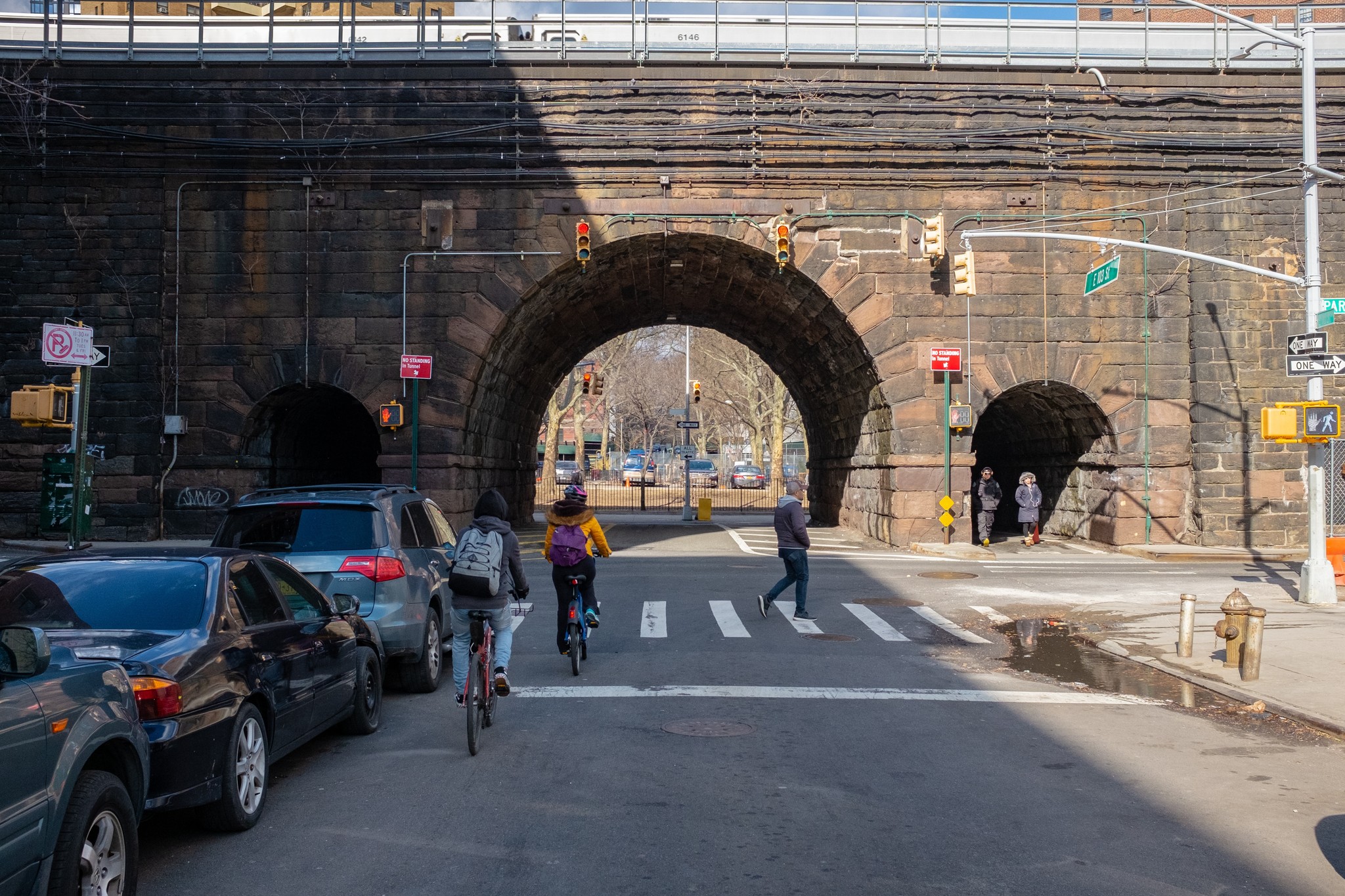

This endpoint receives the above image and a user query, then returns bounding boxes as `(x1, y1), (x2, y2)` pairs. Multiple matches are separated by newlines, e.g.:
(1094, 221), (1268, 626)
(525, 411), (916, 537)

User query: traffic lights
(923, 215), (946, 257)
(380, 403), (403, 427)
(38, 385), (67, 423)
(953, 251), (977, 297)
(574, 221), (590, 261)
(774, 222), (790, 263)
(581, 373), (590, 395)
(949, 405), (972, 427)
(593, 375), (604, 395)
(693, 382), (700, 401)
(1303, 404), (1340, 439)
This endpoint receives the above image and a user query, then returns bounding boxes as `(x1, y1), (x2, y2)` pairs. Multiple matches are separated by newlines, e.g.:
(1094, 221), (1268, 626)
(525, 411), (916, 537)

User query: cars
(667, 445), (681, 454)
(621, 446), (661, 486)
(0, 548), (382, 895)
(537, 461), (591, 484)
(727, 465), (765, 488)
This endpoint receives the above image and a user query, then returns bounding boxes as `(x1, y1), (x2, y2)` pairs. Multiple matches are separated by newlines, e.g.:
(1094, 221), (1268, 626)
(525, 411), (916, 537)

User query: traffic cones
(536, 477), (542, 481)
(625, 477), (630, 487)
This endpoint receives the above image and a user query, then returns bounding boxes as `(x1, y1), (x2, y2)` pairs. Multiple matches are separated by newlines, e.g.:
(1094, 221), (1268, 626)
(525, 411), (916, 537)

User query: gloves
(512, 587), (529, 601)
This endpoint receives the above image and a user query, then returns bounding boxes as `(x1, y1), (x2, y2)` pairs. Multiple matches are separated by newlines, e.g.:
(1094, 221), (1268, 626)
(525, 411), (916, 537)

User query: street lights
(1229, 39), (1337, 603)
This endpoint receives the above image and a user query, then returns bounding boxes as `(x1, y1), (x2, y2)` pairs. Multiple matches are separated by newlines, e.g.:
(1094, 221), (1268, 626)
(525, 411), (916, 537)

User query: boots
(1028, 531), (1034, 545)
(1024, 536), (1031, 546)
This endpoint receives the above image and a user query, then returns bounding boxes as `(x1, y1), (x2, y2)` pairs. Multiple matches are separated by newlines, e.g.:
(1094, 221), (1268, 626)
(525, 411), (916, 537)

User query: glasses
(983, 473), (991, 475)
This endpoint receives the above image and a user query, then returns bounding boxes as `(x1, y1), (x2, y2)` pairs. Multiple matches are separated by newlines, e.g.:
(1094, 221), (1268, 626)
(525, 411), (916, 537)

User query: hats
(983, 468), (992, 473)
(786, 481), (809, 494)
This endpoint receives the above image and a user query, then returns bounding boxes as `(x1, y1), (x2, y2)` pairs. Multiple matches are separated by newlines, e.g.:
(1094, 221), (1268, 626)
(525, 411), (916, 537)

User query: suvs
(207, 485), (461, 693)
(680, 458), (718, 487)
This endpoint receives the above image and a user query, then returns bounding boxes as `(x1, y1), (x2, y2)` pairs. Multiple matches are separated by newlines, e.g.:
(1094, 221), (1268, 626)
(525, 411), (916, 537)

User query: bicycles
(544, 549), (609, 676)
(455, 585), (528, 755)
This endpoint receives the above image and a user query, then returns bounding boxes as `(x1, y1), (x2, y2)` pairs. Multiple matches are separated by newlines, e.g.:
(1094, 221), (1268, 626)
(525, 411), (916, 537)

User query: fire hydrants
(1212, 586), (1253, 668)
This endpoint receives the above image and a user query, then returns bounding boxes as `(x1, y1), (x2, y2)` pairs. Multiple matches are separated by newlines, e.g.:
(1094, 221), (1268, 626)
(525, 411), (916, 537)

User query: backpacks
(550, 524), (589, 567)
(445, 526), (503, 597)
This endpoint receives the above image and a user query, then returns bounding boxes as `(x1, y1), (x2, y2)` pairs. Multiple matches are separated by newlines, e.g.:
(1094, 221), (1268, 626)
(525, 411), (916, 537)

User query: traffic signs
(400, 355), (431, 379)
(1287, 334), (1328, 355)
(930, 347), (962, 372)
(675, 422), (700, 429)
(680, 445), (695, 460)
(1285, 354), (1345, 378)
(45, 345), (111, 369)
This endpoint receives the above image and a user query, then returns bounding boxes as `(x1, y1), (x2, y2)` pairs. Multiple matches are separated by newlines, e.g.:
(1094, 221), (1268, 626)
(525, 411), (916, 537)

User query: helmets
(564, 485), (588, 500)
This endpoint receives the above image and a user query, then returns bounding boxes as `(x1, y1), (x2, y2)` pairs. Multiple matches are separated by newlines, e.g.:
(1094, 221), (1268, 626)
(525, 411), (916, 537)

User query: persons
(758, 481), (818, 621)
(540, 485), (612, 653)
(1015, 472), (1041, 547)
(971, 467), (1002, 547)
(447, 490), (528, 709)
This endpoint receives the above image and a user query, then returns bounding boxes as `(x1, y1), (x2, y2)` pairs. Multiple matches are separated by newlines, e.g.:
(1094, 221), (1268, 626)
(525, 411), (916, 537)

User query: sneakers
(793, 610), (817, 621)
(455, 692), (467, 709)
(494, 666), (511, 697)
(983, 538), (989, 547)
(758, 595), (773, 619)
(585, 608), (600, 629)
